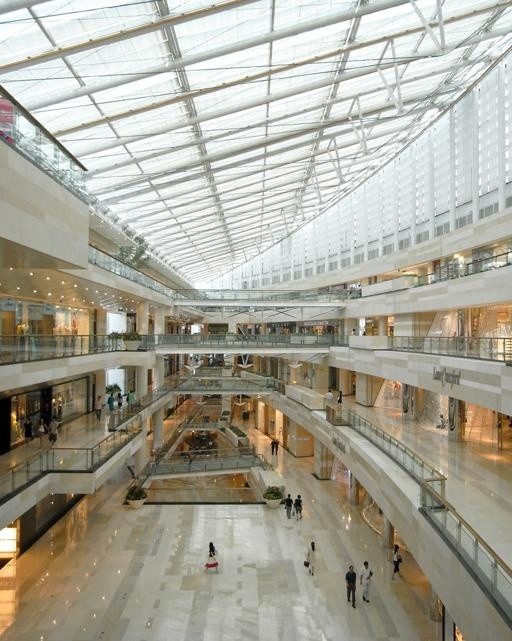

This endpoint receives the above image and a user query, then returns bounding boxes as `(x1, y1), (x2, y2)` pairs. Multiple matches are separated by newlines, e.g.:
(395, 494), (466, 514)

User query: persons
(270, 439), (275, 455)
(336, 391), (343, 404)
(436, 415), (446, 429)
(204, 542), (218, 571)
(392, 545), (403, 580)
(345, 566), (356, 609)
(275, 439), (279, 455)
(94, 391), (123, 422)
(326, 390), (334, 400)
(305, 541), (317, 576)
(294, 495), (302, 522)
(359, 561), (373, 603)
(24, 406), (63, 447)
(285, 494), (293, 519)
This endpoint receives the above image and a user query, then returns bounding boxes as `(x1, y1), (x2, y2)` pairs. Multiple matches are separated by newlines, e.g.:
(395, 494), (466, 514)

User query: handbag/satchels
(304, 561), (309, 567)
(396, 551), (402, 563)
(293, 504), (295, 515)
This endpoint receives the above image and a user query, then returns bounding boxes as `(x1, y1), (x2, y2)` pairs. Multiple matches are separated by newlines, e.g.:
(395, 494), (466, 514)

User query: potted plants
(125, 485), (149, 509)
(122, 333), (143, 351)
(261, 485), (284, 508)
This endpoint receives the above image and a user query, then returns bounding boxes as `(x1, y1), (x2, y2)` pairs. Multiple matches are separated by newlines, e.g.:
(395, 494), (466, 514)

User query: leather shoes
(347, 596), (369, 607)
